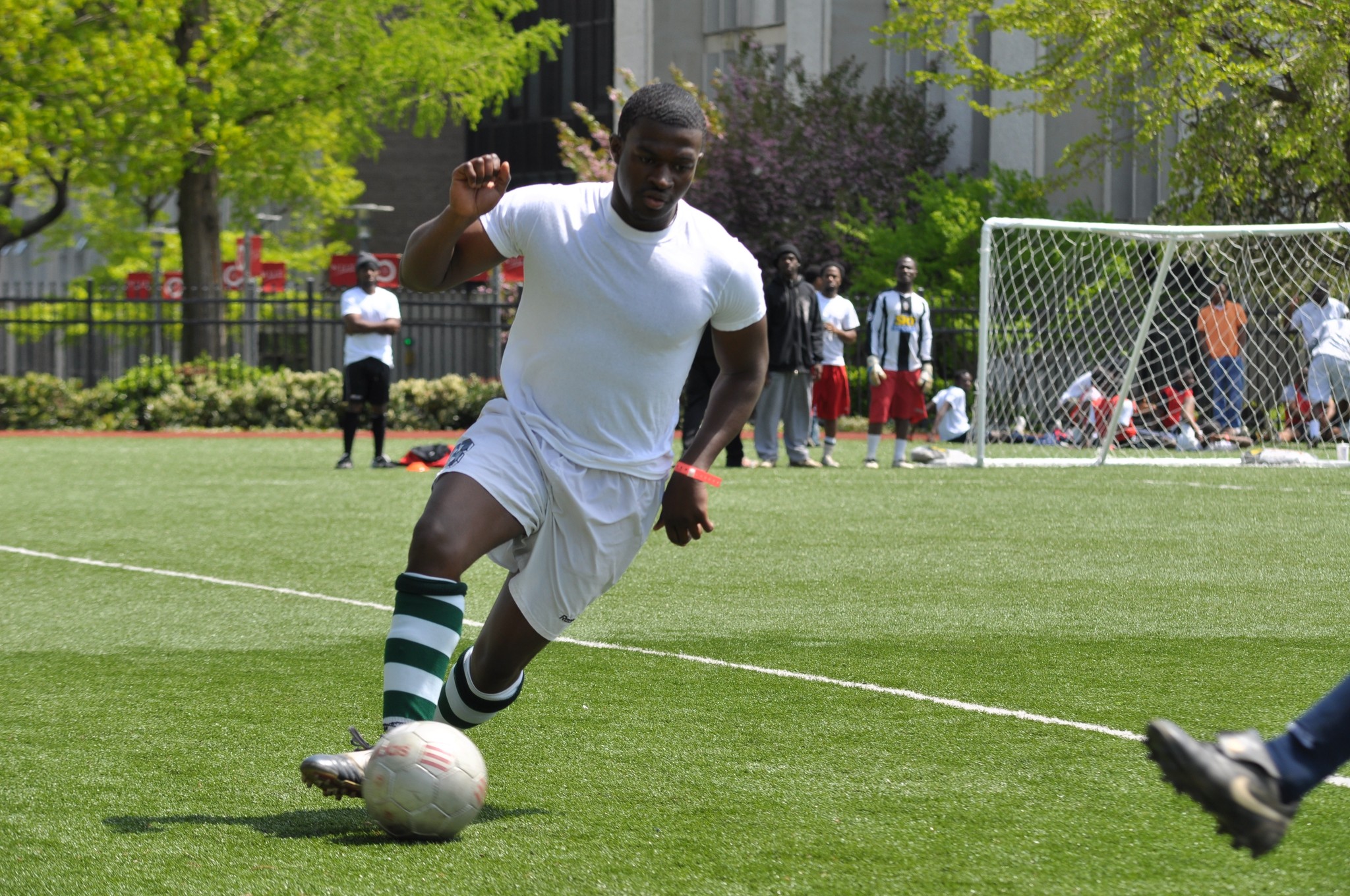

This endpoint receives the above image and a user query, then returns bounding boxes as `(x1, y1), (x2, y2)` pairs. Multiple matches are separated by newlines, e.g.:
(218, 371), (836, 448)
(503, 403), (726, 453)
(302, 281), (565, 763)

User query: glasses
(358, 264), (378, 271)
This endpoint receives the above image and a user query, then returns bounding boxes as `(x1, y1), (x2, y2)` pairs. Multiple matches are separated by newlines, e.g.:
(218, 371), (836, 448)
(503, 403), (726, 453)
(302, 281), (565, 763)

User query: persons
(910, 369), (999, 444)
(335, 255), (401, 468)
(1057, 281), (1350, 450)
(862, 255), (933, 469)
(681, 243), (860, 468)
(297, 84), (772, 803)
(1143, 677), (1350, 860)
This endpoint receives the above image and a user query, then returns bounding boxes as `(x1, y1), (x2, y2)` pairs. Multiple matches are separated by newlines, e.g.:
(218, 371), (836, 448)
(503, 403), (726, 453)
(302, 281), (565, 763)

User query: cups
(1337, 443), (1350, 461)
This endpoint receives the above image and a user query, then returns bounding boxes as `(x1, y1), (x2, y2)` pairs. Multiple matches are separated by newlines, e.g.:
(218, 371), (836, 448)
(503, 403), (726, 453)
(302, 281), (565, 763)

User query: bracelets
(673, 462), (721, 487)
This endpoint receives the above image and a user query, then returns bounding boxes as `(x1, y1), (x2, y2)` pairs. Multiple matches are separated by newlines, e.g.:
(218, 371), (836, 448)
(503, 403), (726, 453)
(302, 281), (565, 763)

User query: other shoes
(1309, 436), (1320, 449)
(864, 458), (878, 469)
(821, 454), (840, 467)
(372, 457), (395, 468)
(789, 458), (823, 468)
(762, 459), (777, 468)
(892, 458), (913, 468)
(741, 456), (759, 469)
(337, 456), (353, 468)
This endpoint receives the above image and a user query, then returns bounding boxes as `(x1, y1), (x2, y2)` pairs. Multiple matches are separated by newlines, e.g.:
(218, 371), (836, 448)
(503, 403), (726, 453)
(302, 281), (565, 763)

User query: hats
(774, 242), (801, 262)
(355, 250), (379, 269)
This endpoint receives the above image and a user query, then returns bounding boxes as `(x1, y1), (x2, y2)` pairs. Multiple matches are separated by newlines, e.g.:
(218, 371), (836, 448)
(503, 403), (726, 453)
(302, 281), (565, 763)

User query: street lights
(338, 203), (395, 254)
(241, 212), (284, 369)
(133, 224), (178, 361)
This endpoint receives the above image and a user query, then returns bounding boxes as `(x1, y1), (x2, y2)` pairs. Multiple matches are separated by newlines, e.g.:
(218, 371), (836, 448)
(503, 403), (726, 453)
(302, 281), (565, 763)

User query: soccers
(361, 721), (487, 844)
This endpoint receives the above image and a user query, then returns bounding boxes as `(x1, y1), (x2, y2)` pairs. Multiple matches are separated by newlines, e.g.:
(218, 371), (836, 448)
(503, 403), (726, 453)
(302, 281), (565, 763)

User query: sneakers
(1143, 719), (1299, 861)
(301, 725), (377, 800)
